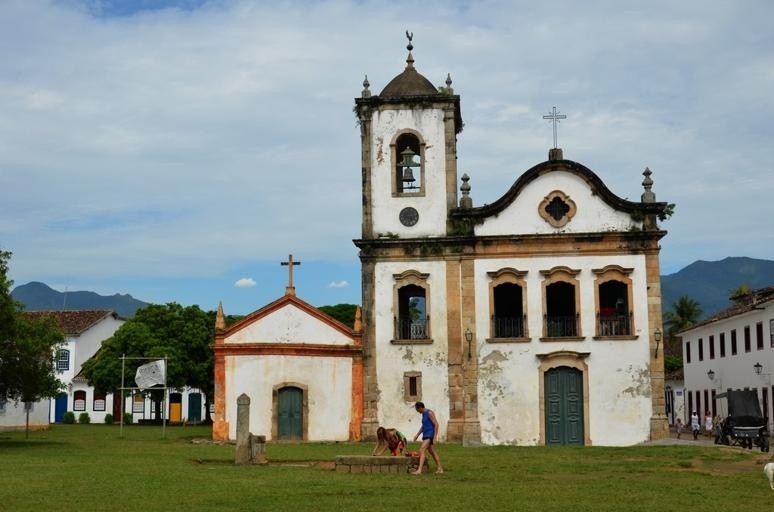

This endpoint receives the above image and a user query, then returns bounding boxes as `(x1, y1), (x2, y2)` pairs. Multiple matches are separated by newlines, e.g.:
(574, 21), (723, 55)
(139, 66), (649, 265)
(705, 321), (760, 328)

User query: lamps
(706, 368), (715, 381)
(752, 362), (766, 376)
(652, 327), (662, 359)
(463, 326), (474, 359)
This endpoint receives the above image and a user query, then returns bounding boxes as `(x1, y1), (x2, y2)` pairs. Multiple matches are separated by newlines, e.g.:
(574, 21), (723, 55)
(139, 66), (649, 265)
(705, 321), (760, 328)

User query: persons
(411, 401), (443, 475)
(703, 411), (713, 440)
(714, 414), (725, 438)
(687, 410), (700, 440)
(675, 418), (683, 438)
(372, 427), (407, 456)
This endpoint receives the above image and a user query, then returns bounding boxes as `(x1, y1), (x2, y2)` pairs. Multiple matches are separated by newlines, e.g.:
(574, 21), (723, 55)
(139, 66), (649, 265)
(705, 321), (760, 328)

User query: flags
(135, 359), (165, 393)
